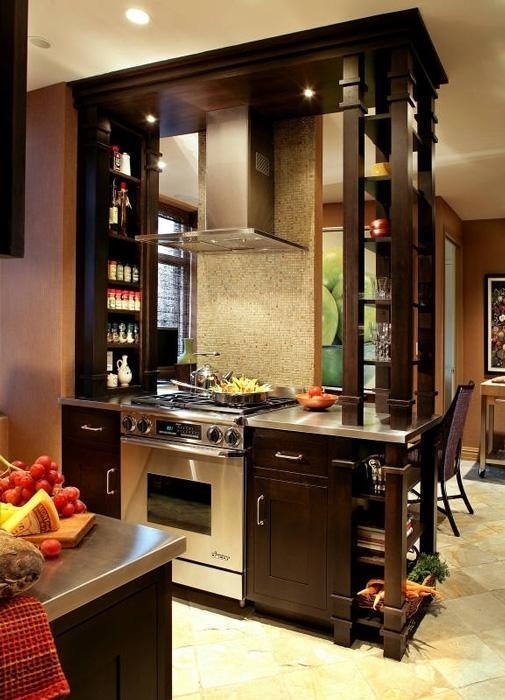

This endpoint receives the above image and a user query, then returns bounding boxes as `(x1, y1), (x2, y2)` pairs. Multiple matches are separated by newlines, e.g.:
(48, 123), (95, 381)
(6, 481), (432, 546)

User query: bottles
(107, 320), (139, 344)
(106, 288), (140, 311)
(109, 178), (130, 237)
(110, 145), (132, 176)
(107, 259), (139, 283)
(176, 335), (196, 363)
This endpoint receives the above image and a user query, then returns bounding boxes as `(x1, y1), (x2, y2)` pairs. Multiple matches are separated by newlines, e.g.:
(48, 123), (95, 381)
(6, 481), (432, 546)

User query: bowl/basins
(294, 393), (340, 409)
(369, 217), (392, 238)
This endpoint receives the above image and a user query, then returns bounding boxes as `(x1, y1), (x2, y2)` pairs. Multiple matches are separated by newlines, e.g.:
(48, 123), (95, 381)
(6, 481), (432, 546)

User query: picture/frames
(483, 272), (504, 377)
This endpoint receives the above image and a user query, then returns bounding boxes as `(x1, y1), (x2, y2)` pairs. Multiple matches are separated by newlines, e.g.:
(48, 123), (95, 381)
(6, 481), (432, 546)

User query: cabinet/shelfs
(59, 400), (121, 519)
(340, 43), (440, 427)
(74, 94), (162, 396)
(245, 430), (439, 659)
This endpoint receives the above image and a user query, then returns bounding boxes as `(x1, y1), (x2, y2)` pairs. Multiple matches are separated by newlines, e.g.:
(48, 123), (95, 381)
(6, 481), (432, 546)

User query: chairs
(407, 380), (476, 537)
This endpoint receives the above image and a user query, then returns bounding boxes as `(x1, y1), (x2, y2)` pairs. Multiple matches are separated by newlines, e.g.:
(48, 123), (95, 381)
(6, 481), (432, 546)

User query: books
(356, 504), (416, 554)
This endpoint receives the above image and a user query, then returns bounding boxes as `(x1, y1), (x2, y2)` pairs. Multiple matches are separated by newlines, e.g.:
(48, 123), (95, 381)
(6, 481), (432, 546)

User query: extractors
(135, 104), (308, 256)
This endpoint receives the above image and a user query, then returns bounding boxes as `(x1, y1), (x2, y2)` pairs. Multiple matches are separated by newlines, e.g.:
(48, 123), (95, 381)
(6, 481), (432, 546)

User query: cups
(106, 373), (118, 388)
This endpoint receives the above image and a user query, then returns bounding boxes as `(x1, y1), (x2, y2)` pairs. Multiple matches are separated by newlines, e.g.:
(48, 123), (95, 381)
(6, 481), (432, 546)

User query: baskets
(358, 558), (434, 619)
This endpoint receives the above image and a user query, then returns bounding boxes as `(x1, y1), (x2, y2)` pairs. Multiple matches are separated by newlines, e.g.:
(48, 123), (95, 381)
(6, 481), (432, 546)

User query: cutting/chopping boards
(1, 507), (99, 551)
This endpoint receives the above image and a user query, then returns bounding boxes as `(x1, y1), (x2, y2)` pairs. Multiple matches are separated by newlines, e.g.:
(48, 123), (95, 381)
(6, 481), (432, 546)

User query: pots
(213, 390), (268, 406)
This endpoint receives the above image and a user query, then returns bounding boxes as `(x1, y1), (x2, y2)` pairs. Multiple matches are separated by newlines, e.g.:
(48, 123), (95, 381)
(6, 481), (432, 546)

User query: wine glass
(369, 275), (393, 300)
(368, 321), (391, 362)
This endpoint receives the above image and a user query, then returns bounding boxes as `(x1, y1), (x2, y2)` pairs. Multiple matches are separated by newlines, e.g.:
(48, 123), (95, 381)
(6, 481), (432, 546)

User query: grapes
(0, 455), (86, 516)
(41, 539), (62, 556)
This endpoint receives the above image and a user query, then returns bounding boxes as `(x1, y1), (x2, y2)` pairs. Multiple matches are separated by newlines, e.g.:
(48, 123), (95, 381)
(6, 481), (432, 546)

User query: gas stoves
(120, 384), (296, 449)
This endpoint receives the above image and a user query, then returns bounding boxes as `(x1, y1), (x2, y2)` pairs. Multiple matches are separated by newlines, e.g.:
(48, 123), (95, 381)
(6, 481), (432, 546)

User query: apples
(309, 386), (321, 396)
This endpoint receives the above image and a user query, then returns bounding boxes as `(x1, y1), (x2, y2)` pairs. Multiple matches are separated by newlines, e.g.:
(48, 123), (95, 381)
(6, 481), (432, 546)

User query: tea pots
(189, 362), (234, 397)
(116, 354), (134, 386)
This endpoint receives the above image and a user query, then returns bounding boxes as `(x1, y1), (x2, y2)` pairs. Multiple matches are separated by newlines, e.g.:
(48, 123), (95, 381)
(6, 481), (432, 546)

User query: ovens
(119, 437), (244, 576)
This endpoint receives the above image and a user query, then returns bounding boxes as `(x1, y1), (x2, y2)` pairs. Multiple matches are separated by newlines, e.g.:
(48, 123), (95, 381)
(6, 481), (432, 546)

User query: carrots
(357, 577), (448, 609)
